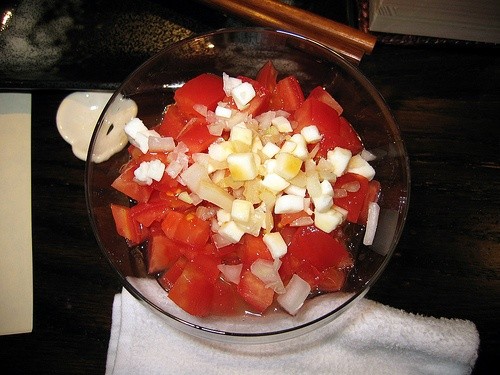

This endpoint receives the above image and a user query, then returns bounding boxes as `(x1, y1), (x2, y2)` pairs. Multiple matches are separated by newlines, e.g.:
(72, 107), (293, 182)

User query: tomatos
(109, 62), (382, 320)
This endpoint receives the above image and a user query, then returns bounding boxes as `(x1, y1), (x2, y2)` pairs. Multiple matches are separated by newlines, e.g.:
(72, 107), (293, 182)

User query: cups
(84, 27), (411, 343)
